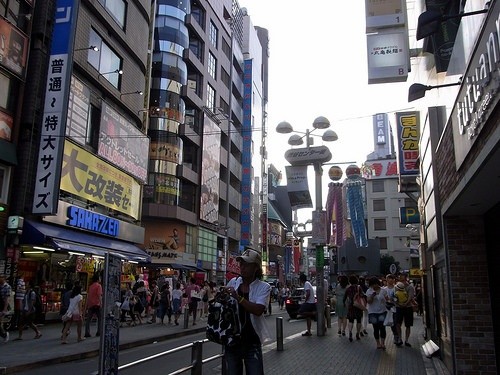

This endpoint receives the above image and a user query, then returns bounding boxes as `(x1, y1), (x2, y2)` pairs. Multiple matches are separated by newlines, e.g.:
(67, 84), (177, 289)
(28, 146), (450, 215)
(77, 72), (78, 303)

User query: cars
(285, 286), (337, 319)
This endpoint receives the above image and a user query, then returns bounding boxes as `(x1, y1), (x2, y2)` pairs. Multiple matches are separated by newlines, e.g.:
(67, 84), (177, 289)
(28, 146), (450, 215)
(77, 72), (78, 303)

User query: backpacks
(205, 290), (241, 346)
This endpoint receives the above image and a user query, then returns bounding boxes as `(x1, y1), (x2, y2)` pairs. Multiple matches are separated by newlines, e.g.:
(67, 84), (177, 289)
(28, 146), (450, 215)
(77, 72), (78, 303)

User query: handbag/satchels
(121, 296), (130, 310)
(130, 296), (137, 305)
(190, 285), (201, 301)
(353, 284), (366, 311)
(166, 306), (173, 316)
(6, 292), (15, 315)
(62, 311), (73, 321)
(383, 309), (394, 326)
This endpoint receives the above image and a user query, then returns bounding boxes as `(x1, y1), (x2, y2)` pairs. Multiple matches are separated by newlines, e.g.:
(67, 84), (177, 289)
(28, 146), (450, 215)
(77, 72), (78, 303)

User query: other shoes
(61, 341), (68, 344)
(77, 338), (86, 342)
(96, 332), (101, 337)
(34, 334), (43, 339)
(84, 334), (91, 338)
(302, 331), (312, 336)
(14, 337), (22, 341)
(120, 314), (209, 328)
(337, 327), (412, 351)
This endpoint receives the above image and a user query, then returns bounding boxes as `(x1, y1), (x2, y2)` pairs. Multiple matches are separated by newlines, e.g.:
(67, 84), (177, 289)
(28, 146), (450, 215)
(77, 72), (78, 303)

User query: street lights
(275, 116), (339, 336)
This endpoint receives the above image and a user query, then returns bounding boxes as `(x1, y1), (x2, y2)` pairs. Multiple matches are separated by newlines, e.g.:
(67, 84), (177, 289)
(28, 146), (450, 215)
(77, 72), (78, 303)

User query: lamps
(416, 10), (488, 40)
(207, 220), (231, 231)
(74, 46), (99, 51)
(98, 69), (124, 77)
(138, 108), (160, 112)
(208, 107), (234, 122)
(120, 90), (144, 96)
(408, 83), (461, 102)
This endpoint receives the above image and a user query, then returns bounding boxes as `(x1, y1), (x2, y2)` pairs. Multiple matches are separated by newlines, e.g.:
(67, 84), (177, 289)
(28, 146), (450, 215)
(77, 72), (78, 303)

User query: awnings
(21, 219), (152, 264)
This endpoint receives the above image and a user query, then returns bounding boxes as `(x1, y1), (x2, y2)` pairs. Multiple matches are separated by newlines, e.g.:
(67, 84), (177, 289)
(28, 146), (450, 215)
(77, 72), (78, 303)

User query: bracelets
(239, 298), (244, 303)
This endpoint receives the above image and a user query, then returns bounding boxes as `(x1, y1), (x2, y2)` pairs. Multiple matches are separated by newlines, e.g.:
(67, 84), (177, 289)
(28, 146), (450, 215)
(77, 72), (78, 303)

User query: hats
(395, 282), (405, 290)
(236, 249), (261, 266)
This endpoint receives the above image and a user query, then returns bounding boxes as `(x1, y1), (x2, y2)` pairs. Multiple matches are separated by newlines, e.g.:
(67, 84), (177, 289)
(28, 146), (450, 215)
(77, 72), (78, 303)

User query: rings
(228, 289), (231, 291)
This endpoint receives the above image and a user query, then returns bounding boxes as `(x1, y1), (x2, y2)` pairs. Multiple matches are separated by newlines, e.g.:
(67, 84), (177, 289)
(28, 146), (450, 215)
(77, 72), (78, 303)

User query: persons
(226, 249), (272, 375)
(0, 273), (220, 344)
(7, 35), (24, 67)
(271, 273), (422, 350)
(166, 229), (179, 249)
